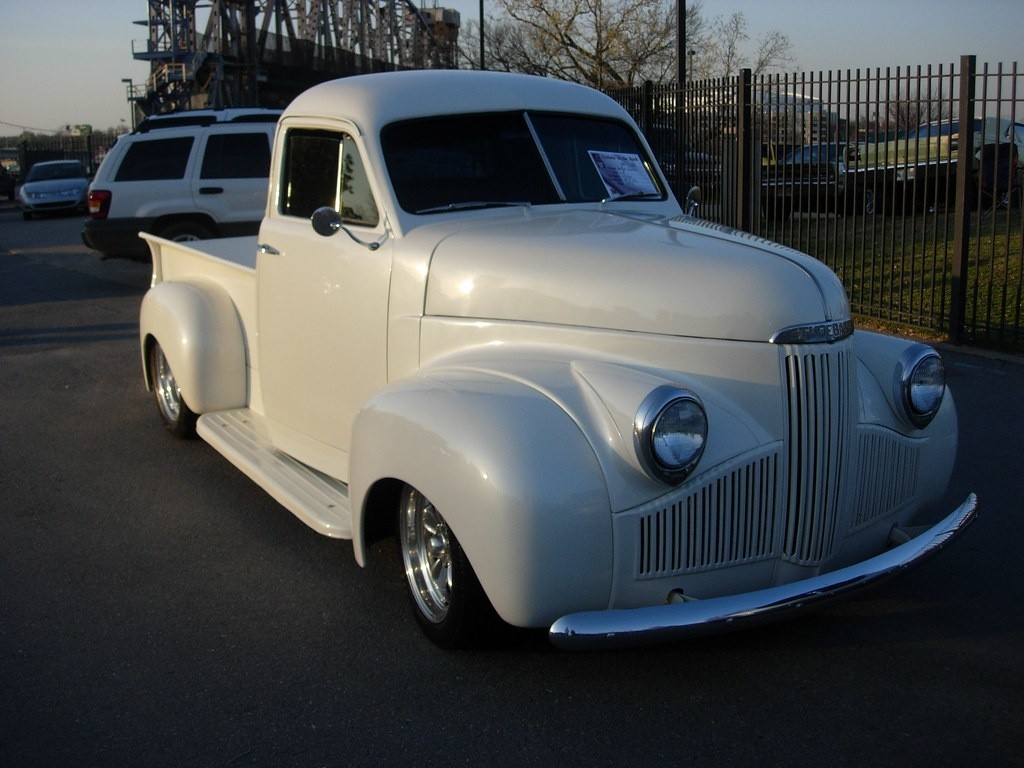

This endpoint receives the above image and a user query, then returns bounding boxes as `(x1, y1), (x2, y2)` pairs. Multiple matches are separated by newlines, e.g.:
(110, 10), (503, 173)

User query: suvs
(82, 106), (287, 262)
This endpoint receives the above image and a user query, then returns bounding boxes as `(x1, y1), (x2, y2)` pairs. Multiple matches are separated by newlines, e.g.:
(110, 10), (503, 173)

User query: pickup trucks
(140, 70), (986, 657)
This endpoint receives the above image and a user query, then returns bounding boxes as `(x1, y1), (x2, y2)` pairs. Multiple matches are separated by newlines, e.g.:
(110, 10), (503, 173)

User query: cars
(19, 159), (89, 220)
(0, 164), (18, 202)
(640, 123), (722, 202)
(841, 114), (1023, 212)
(761, 139), (881, 217)
